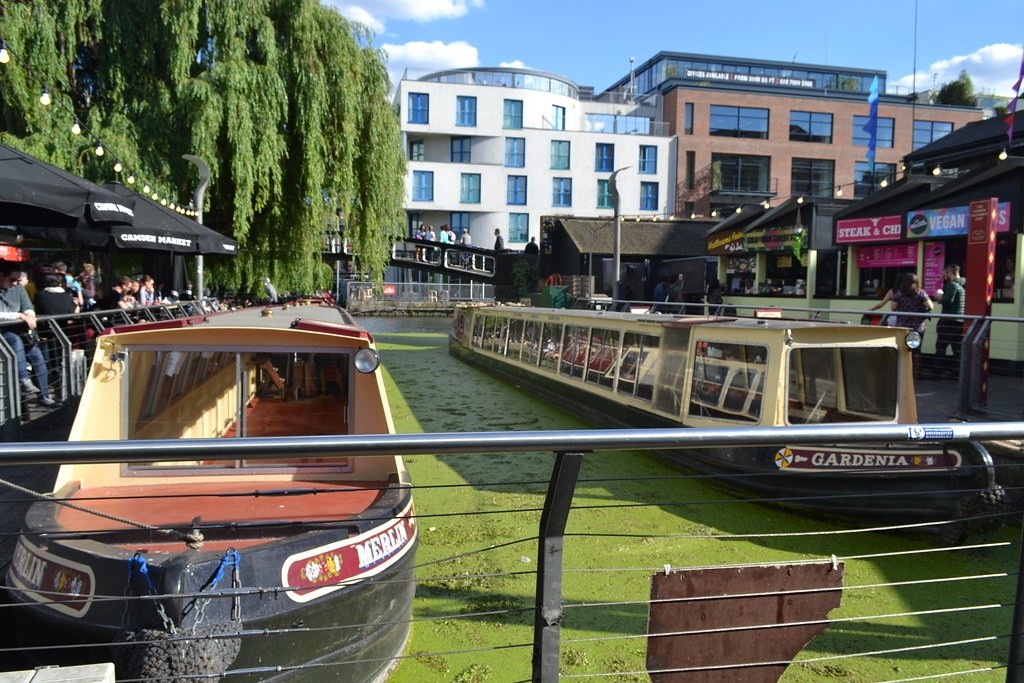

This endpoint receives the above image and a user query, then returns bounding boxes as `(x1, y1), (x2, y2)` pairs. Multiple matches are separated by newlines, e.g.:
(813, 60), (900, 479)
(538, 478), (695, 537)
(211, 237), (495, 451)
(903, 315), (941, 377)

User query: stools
(428, 289), (450, 302)
(364, 288), (372, 300)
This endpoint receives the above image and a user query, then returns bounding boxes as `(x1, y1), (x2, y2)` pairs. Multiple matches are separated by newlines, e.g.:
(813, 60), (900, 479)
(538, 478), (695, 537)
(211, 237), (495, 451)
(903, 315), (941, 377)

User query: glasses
(6, 275), (22, 283)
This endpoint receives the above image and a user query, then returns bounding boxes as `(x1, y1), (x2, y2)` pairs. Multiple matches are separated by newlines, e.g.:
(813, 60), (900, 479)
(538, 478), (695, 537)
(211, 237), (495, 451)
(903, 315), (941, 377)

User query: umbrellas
(0, 143), (237, 267)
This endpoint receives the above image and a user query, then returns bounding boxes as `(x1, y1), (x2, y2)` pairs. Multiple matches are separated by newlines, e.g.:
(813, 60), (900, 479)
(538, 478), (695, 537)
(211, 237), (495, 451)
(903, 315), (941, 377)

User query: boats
(0, 284), (423, 683)
(449, 302), (1007, 549)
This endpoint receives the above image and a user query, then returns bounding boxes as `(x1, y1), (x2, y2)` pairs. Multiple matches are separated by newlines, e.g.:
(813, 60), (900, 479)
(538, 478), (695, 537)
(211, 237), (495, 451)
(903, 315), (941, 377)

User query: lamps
(905, 331), (922, 351)
(354, 348), (382, 373)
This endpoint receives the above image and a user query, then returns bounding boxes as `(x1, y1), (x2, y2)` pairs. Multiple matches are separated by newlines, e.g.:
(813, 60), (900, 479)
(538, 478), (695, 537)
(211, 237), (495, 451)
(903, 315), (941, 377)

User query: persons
(932, 263), (965, 381)
(0, 262), (196, 413)
(263, 278), (277, 303)
(439, 224), (471, 270)
(524, 237), (538, 255)
(869, 272), (934, 381)
(416, 224), (436, 263)
(494, 228), (504, 250)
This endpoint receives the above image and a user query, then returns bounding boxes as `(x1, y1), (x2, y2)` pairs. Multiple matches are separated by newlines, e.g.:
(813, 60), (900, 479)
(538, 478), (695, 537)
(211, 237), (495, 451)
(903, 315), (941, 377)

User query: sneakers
(21, 379), (40, 394)
(37, 395), (55, 405)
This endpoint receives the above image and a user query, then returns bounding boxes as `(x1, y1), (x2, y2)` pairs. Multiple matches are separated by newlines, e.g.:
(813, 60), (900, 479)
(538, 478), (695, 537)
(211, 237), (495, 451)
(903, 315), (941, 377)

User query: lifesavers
(545, 276), (553, 285)
(557, 273), (562, 286)
(552, 274), (558, 286)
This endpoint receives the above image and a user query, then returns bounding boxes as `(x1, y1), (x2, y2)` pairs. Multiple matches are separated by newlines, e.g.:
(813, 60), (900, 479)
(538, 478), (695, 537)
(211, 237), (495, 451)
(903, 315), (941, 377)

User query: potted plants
(511, 257), (532, 306)
